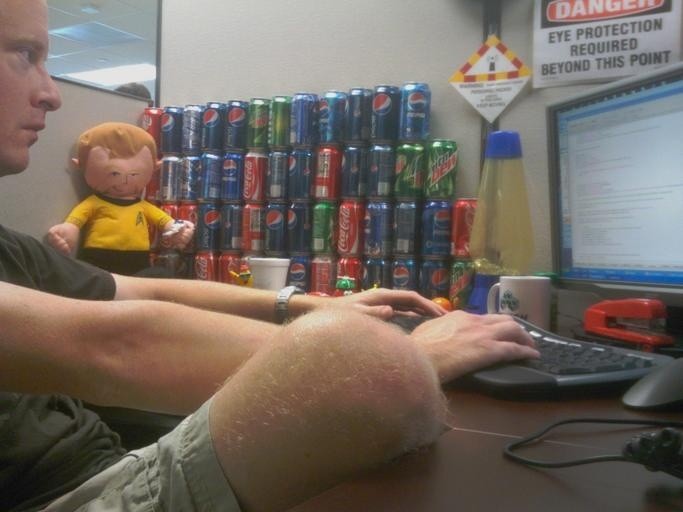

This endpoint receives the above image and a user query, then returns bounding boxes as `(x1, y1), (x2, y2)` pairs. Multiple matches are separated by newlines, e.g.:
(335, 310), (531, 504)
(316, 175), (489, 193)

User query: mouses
(622, 358), (683, 408)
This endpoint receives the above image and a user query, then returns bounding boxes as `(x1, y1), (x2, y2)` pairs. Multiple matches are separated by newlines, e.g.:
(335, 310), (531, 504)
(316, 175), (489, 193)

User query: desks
(284, 386), (683, 512)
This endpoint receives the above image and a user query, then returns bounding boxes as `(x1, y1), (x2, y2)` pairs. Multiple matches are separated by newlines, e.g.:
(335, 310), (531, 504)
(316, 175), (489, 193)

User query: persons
(0, 1), (539, 512)
(45, 121), (194, 275)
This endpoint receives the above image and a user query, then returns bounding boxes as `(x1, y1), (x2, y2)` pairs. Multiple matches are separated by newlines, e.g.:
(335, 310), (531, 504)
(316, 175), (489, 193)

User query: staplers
(584, 298), (676, 348)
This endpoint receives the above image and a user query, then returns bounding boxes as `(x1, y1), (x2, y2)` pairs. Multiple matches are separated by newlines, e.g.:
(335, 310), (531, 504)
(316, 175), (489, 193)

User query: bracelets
(274, 284), (303, 323)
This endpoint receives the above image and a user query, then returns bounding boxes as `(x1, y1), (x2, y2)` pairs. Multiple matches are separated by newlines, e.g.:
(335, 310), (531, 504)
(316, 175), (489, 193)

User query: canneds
(138, 80), (479, 311)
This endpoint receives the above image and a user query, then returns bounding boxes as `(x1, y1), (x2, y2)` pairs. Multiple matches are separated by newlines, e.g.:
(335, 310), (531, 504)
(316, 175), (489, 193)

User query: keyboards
(386, 311), (674, 403)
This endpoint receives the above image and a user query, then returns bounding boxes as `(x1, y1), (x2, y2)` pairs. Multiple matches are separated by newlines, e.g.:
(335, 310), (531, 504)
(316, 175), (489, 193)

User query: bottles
(470, 129), (534, 314)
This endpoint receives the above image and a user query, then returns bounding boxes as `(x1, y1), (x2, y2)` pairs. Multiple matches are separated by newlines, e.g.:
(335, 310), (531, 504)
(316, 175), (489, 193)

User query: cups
(243, 257), (291, 291)
(486, 276), (554, 333)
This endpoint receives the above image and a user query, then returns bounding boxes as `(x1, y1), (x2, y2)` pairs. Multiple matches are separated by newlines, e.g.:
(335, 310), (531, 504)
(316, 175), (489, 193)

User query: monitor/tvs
(545, 62), (683, 359)
